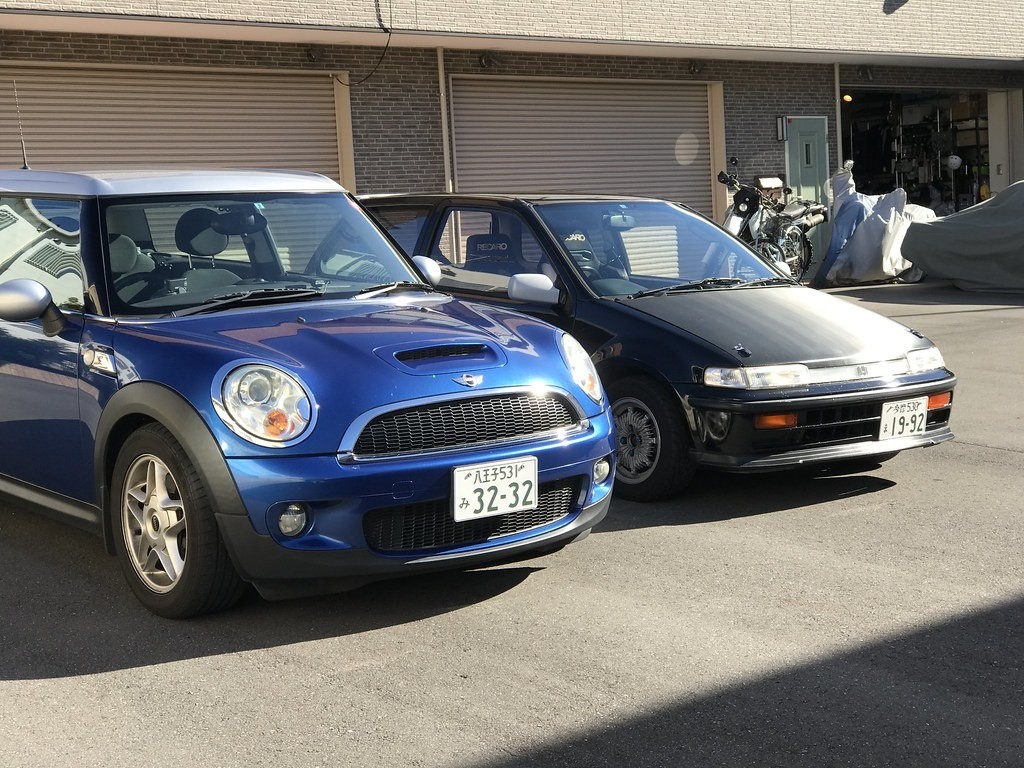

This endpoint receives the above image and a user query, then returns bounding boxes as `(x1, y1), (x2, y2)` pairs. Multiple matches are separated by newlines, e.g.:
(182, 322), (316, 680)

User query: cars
(0, 166), (618, 620)
(304, 191), (959, 508)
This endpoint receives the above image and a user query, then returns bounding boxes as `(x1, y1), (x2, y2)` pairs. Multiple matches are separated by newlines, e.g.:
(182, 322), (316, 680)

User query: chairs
(105, 232), (167, 304)
(463, 233), (529, 277)
(538, 235), (649, 294)
(173, 208), (246, 293)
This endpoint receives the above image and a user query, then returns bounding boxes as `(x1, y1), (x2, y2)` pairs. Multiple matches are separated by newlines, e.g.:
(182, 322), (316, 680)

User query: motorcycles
(698, 157), (827, 281)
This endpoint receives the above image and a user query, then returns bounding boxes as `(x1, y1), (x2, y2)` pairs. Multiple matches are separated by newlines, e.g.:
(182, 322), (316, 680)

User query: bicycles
(741, 186), (813, 286)
